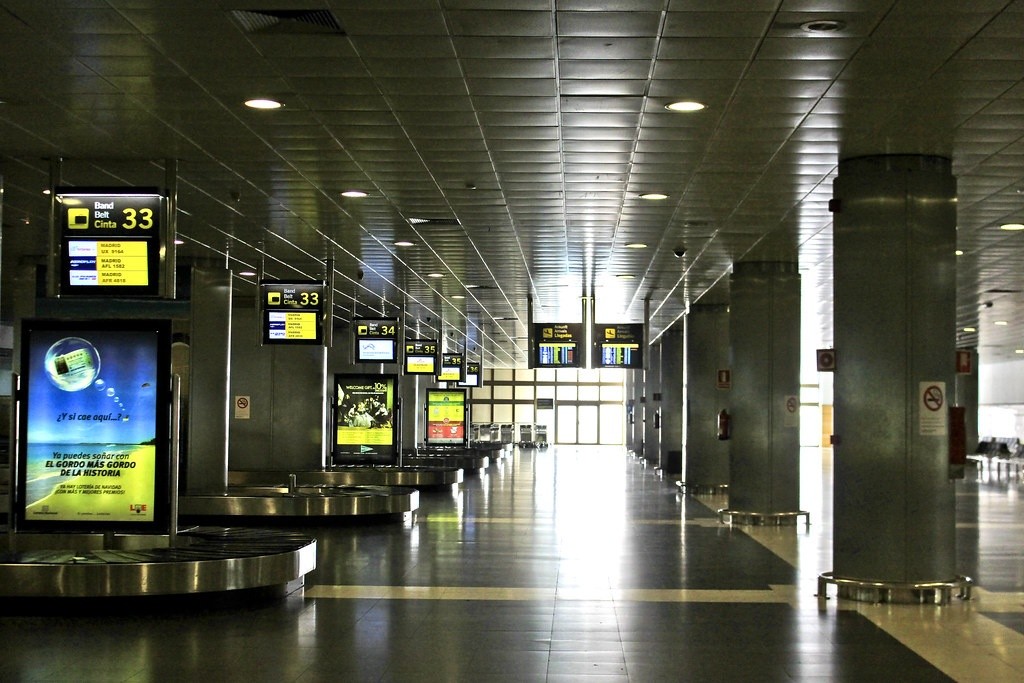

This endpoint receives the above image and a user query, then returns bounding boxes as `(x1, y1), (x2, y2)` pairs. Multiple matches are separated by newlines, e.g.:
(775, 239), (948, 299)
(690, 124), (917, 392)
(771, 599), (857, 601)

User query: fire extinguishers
(653, 413), (659, 428)
(716, 409), (730, 440)
(949, 406), (968, 466)
